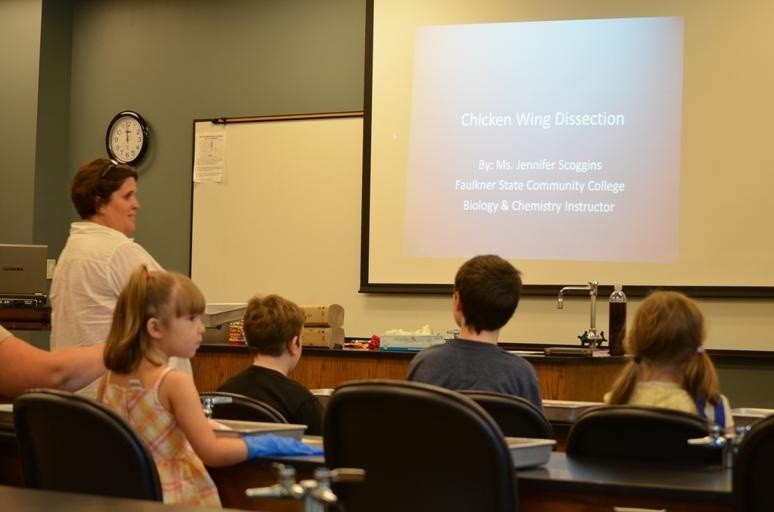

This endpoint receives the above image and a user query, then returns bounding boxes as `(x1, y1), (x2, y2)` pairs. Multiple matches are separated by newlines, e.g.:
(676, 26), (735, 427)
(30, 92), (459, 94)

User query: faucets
(557, 281), (605, 347)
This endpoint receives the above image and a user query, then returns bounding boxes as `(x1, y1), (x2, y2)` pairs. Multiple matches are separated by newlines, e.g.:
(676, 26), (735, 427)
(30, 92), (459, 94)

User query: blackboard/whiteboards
(189, 112), (774, 369)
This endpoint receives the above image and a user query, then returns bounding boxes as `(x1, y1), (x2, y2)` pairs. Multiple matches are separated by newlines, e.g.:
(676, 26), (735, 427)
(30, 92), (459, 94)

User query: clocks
(103, 108), (151, 167)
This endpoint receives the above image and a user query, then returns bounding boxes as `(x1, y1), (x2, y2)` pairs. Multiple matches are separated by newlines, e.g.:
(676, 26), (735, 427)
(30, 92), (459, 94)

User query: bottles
(607, 283), (628, 355)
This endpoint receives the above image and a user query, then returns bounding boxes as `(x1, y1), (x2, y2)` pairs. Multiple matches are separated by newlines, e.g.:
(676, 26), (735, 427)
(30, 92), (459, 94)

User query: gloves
(243, 433), (325, 463)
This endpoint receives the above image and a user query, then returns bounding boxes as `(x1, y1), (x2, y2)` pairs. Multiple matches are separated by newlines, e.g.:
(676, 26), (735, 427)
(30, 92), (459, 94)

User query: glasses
(100, 159), (119, 178)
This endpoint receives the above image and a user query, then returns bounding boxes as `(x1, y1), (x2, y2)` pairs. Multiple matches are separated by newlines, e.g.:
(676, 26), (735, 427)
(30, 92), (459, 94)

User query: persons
(96, 265), (324, 511)
(47, 158), (195, 402)
(211, 294), (325, 436)
(404, 256), (544, 415)
(0, 321), (111, 404)
(601, 289), (735, 436)
